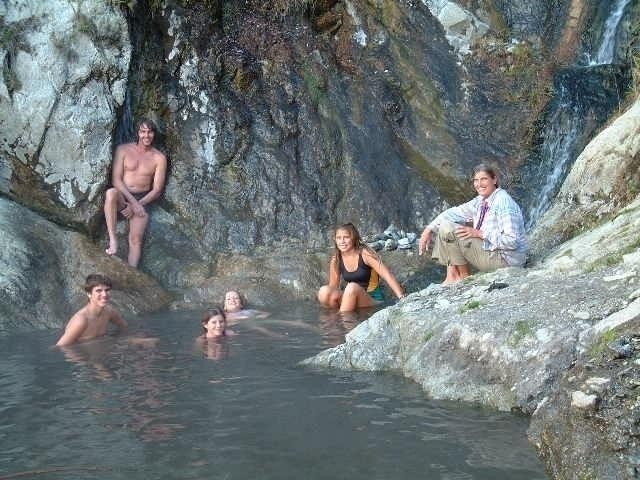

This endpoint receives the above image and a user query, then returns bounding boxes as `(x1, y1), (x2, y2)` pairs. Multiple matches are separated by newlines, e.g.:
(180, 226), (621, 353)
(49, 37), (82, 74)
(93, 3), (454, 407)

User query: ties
(474, 205), (487, 230)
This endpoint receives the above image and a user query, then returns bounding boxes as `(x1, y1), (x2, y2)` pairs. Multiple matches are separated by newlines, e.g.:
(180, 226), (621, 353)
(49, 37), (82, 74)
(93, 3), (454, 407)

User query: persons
(221, 288), (325, 344)
(191, 305), (234, 343)
(103, 116), (168, 271)
(417, 163), (528, 284)
(55, 270), (128, 345)
(317, 219), (409, 318)
